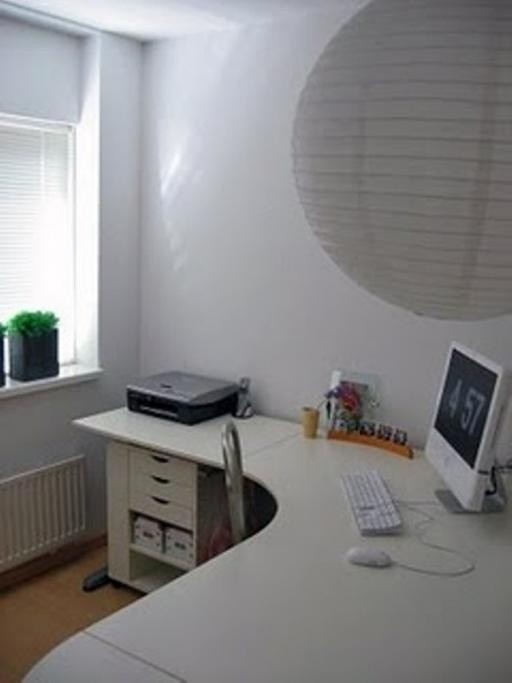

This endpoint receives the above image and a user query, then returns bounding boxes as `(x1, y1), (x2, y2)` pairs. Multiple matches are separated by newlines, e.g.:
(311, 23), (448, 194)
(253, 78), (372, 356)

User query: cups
(300, 406), (319, 439)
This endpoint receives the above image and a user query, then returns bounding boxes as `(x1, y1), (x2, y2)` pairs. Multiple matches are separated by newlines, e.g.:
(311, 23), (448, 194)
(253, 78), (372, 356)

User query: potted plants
(0, 309), (61, 388)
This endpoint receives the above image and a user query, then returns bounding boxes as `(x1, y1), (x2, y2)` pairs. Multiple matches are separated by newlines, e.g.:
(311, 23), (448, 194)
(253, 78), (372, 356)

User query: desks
(18, 393), (512, 683)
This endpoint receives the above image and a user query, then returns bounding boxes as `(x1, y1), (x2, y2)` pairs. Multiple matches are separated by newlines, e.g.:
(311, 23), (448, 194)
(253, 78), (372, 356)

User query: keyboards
(337, 468), (404, 536)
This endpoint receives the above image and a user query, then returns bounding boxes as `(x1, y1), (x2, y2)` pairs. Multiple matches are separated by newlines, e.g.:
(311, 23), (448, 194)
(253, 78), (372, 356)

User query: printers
(125, 369), (240, 426)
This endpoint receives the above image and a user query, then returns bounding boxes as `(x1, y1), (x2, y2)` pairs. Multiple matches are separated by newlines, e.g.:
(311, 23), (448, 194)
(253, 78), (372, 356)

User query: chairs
(219, 415), (251, 545)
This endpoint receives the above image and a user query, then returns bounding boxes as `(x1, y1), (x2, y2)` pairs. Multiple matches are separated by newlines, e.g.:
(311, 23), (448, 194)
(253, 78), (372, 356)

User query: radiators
(0, 454), (90, 575)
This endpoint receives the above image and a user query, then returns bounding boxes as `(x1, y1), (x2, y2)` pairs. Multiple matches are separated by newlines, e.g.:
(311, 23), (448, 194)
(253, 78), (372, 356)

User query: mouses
(346, 546), (391, 567)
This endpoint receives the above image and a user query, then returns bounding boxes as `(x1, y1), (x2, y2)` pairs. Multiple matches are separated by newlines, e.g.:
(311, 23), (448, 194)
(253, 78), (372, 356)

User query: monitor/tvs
(423, 340), (505, 514)
